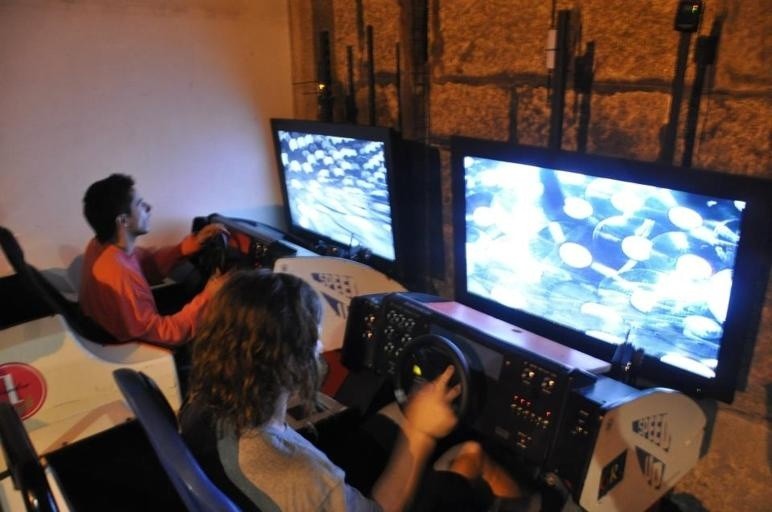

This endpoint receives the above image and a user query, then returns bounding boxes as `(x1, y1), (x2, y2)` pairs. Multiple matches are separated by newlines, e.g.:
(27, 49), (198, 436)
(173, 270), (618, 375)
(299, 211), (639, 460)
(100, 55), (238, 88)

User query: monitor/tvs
(449, 135), (772, 405)
(271, 117), (407, 272)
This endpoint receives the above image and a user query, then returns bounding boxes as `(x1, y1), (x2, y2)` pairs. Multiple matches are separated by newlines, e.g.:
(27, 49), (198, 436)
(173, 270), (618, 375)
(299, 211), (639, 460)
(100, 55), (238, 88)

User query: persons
(178, 269), (523, 511)
(76, 173), (241, 352)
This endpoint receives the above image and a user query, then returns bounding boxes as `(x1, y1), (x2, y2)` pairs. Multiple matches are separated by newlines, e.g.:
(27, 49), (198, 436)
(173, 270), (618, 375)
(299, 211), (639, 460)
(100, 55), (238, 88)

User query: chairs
(0, 228), (122, 345)
(111, 368), (247, 512)
